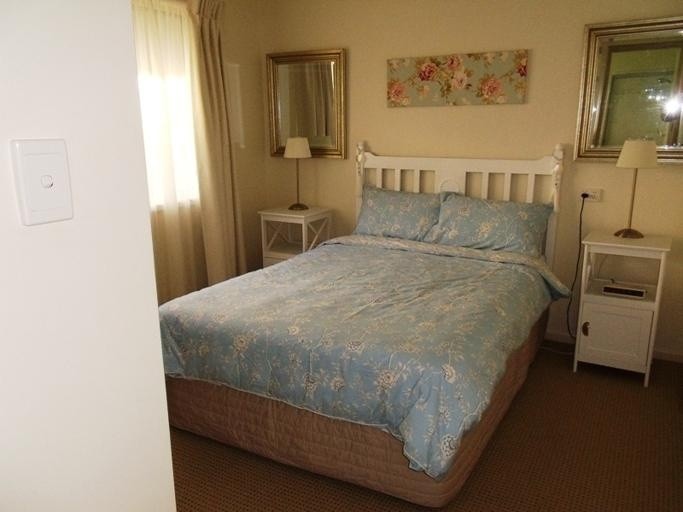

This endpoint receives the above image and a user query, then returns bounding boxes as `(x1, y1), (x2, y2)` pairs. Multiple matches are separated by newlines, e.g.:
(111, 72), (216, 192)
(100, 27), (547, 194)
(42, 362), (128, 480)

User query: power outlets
(579, 187), (602, 203)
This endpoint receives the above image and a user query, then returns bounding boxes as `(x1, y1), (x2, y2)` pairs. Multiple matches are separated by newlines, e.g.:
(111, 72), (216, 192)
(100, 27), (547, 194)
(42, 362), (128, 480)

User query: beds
(158, 139), (565, 508)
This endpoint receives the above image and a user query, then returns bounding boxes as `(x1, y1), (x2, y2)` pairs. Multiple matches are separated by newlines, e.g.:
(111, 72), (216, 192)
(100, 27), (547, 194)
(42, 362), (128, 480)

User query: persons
(658, 91), (682, 147)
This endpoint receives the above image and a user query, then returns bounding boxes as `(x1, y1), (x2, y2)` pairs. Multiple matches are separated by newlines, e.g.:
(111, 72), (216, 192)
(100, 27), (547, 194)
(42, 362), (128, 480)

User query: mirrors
(264, 48), (347, 159)
(573, 17), (683, 163)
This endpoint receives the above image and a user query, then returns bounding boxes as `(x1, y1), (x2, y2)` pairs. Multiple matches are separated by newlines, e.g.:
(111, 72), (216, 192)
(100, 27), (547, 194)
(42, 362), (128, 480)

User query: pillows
(352, 184), (440, 240)
(424, 191), (553, 260)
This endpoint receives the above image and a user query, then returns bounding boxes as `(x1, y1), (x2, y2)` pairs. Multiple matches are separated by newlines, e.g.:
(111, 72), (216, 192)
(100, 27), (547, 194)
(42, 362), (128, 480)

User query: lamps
(283, 135), (311, 211)
(614, 137), (658, 237)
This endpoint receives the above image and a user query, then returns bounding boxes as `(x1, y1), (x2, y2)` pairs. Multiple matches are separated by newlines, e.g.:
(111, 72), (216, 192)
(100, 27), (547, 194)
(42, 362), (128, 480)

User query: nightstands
(258, 205), (335, 268)
(573, 229), (673, 388)
